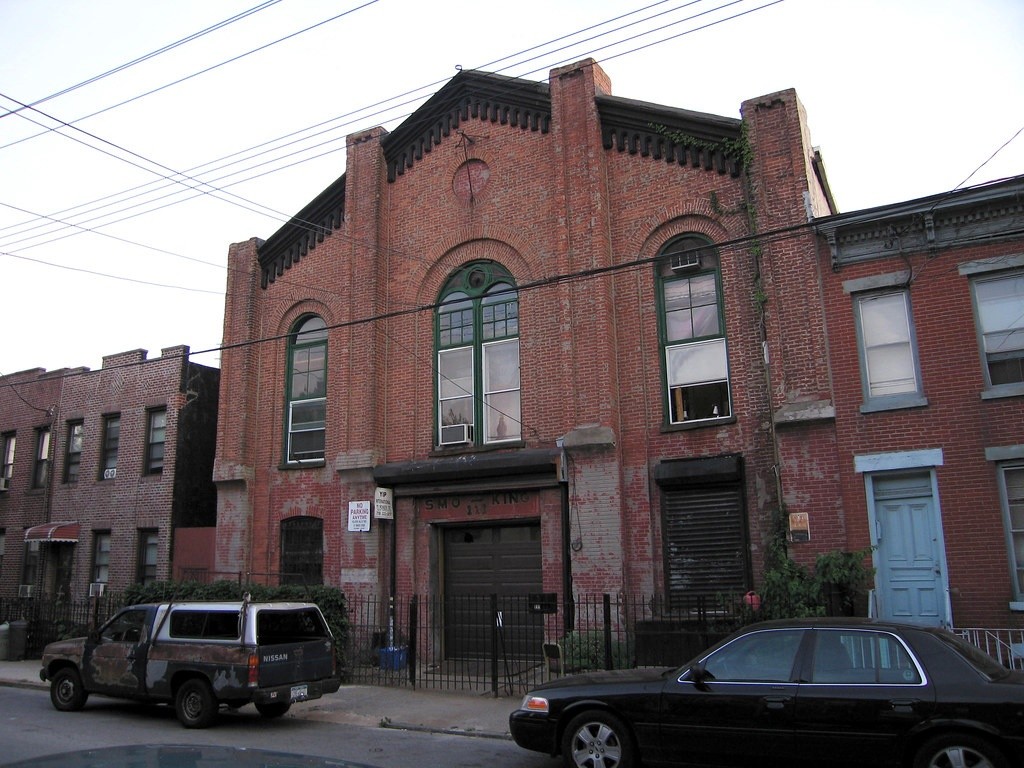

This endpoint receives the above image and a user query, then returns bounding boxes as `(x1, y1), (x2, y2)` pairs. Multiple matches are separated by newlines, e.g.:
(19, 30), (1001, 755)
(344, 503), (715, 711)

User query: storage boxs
(379, 647), (406, 671)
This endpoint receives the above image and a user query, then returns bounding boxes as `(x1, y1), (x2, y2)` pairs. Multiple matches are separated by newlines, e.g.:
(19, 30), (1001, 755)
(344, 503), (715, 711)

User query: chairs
(542, 642), (581, 681)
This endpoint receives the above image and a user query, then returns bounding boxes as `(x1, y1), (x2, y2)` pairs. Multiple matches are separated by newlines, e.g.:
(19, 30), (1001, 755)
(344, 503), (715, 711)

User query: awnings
(21, 520), (80, 543)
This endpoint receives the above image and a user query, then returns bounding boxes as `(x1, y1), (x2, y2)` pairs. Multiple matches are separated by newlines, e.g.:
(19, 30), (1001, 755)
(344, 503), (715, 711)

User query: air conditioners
(90, 583), (109, 596)
(18, 585), (36, 597)
(104, 469), (116, 479)
(0, 478), (10, 490)
(440, 424), (473, 446)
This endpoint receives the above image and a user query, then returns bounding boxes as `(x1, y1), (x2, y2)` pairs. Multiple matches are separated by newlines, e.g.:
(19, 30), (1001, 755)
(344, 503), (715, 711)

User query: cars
(509, 617), (1024, 768)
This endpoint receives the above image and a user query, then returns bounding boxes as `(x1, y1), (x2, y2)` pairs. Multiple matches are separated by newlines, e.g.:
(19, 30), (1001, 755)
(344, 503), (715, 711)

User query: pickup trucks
(39, 602), (341, 728)
